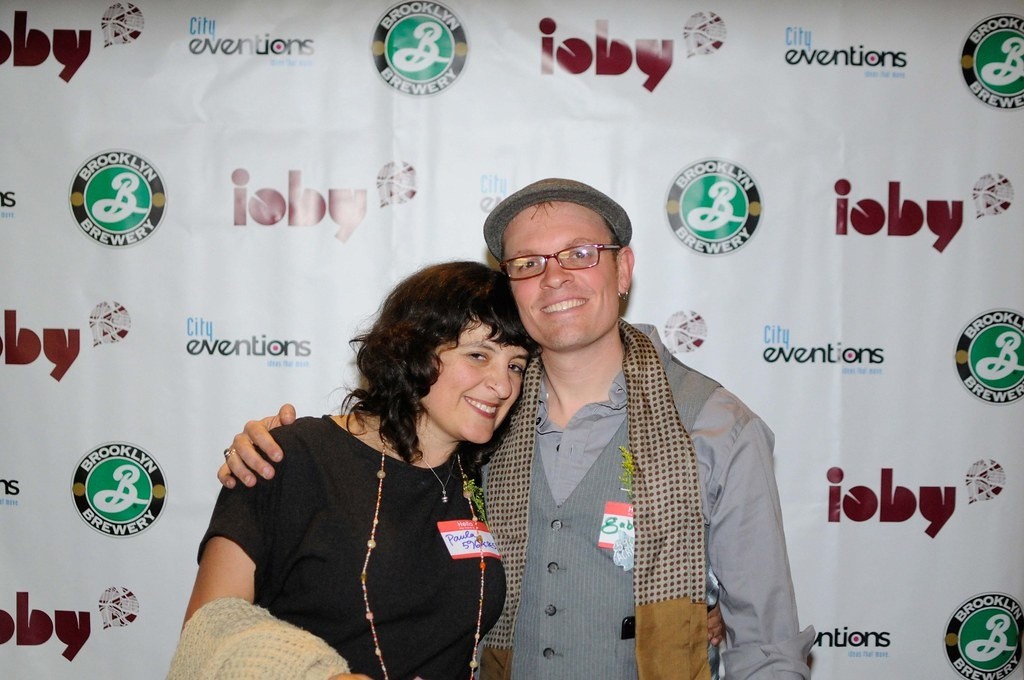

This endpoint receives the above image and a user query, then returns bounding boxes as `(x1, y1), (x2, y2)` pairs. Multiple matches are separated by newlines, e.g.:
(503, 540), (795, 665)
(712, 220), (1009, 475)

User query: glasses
(500, 243), (621, 280)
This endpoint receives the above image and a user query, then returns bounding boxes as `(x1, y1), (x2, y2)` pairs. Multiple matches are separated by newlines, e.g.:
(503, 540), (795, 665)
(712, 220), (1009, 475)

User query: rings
(223, 449), (236, 458)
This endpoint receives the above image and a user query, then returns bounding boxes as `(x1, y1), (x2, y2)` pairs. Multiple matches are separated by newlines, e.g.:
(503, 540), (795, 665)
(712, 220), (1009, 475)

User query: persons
(181, 178), (816, 680)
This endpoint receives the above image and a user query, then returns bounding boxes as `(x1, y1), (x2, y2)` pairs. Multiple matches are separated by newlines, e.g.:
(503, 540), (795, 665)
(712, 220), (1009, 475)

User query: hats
(483, 178), (632, 263)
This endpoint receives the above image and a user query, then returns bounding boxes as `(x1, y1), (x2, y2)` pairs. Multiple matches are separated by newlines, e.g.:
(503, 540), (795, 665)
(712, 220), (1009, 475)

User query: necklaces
(418, 453), (456, 503)
(362, 439), (485, 680)
